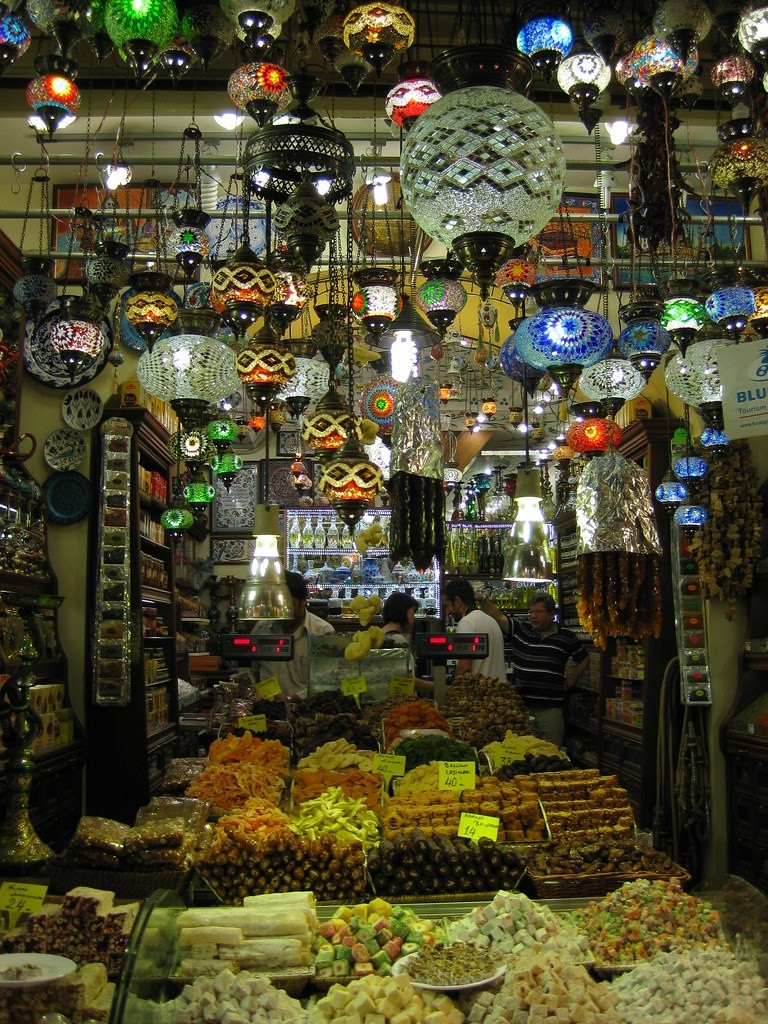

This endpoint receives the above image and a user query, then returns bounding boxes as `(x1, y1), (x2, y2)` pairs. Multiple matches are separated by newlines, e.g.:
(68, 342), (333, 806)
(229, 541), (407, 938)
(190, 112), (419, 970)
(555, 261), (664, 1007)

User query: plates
(391, 946), (508, 990)
(112, 287), (183, 351)
(62, 386), (104, 431)
(24, 295), (113, 389)
(42, 469), (92, 525)
(44, 427), (87, 472)
(183, 282), (212, 309)
(0, 953), (81, 988)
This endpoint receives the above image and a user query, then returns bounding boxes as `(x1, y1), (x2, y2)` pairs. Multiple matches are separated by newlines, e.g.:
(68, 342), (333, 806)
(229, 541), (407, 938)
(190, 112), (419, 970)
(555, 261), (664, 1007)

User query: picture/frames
(52, 184), (201, 284)
(511, 191), (609, 292)
(607, 192), (748, 293)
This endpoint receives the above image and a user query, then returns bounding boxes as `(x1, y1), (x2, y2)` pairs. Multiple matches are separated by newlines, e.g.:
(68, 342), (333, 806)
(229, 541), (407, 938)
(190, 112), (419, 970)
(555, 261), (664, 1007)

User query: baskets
(366, 889), (522, 904)
(52, 853), (196, 900)
(210, 892), (371, 908)
(526, 844), (691, 898)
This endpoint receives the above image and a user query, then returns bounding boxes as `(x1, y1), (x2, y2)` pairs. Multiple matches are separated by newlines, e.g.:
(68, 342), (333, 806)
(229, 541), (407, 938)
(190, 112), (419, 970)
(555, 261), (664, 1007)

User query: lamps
(0, 0), (768, 620)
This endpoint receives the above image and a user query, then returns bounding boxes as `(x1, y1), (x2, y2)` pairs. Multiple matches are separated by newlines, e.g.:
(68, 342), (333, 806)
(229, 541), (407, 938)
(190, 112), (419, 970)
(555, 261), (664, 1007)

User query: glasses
(527, 610), (550, 616)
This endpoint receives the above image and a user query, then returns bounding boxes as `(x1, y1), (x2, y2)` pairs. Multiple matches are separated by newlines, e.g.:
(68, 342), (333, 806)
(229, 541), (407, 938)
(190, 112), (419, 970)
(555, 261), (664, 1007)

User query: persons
(380, 592), (434, 699)
(474, 591), (590, 749)
(440, 577), (508, 683)
(250, 571), (340, 705)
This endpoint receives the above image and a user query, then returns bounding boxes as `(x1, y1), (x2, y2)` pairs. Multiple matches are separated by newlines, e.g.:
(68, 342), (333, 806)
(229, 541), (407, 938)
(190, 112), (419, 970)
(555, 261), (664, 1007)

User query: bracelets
(286, 696), (291, 702)
(565, 680), (568, 689)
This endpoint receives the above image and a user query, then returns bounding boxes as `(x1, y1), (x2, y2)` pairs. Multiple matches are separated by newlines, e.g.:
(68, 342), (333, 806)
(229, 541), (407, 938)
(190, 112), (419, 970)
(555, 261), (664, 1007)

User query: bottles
(286, 514), (438, 619)
(445, 524), (559, 612)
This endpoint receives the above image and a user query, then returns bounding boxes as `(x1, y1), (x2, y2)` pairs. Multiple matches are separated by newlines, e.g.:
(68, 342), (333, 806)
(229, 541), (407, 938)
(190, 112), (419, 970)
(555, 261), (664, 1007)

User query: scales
(411, 631), (489, 715)
(223, 633), (295, 677)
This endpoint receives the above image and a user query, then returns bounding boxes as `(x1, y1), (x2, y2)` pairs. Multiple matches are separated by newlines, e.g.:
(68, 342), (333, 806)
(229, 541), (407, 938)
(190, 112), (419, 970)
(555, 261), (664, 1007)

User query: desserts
(0, 768), (768, 1024)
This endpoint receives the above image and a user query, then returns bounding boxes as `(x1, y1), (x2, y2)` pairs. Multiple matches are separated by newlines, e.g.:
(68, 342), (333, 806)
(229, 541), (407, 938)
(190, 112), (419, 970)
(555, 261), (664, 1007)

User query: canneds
(144, 648), (168, 683)
(146, 686), (168, 734)
(140, 551), (168, 590)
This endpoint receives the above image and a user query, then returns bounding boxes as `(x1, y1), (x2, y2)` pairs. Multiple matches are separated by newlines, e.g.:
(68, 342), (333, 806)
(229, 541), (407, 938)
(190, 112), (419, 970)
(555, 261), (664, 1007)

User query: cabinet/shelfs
(0, 230), (106, 886)
(284, 508), (444, 626)
(445, 516), (560, 640)
(545, 409), (679, 837)
